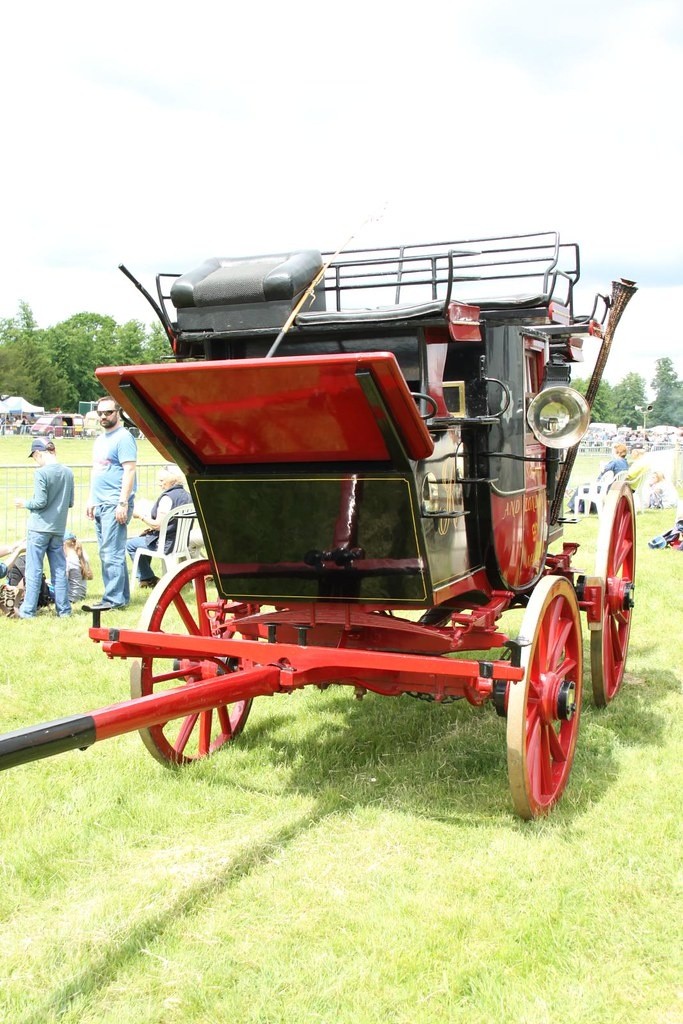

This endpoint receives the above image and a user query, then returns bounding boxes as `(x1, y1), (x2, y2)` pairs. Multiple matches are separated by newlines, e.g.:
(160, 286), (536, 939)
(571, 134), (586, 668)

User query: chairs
(129, 502), (203, 591)
(574, 449), (678, 516)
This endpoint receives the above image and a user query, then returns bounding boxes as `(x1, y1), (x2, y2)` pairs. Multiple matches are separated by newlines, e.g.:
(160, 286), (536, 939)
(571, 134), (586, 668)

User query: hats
(614, 444), (627, 456)
(63, 534), (76, 541)
(28, 437), (52, 457)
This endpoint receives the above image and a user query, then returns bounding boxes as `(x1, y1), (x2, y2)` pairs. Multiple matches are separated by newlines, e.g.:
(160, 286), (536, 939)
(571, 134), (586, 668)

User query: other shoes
(93, 600), (125, 609)
(140, 577), (160, 588)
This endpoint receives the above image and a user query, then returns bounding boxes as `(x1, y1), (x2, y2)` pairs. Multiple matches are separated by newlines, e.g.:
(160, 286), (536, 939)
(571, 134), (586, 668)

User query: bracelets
(141, 515), (146, 521)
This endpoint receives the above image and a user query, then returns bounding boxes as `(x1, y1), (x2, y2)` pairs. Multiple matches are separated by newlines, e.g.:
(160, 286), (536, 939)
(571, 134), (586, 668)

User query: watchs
(118, 500), (128, 507)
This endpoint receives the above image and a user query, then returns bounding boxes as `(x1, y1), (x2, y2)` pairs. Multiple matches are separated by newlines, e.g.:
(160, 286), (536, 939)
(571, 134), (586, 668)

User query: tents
(0, 396), (45, 424)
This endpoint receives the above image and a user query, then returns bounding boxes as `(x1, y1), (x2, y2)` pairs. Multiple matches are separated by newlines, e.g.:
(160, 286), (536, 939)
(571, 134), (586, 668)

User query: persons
(625, 444), (649, 493)
(14, 437), (74, 619)
(0, 538), (26, 579)
(648, 471), (679, 509)
(564, 444), (629, 515)
(44, 530), (93, 603)
(82, 396), (137, 612)
(62, 421), (69, 437)
(578, 429), (683, 453)
(126, 470), (194, 588)
(0, 414), (35, 435)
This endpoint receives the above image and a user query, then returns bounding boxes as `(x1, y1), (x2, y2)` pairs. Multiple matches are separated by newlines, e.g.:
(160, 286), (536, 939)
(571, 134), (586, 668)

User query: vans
(29, 412), (85, 439)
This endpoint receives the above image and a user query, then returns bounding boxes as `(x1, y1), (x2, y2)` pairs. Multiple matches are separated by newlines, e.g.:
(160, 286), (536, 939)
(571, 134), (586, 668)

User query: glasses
(97, 410), (117, 416)
